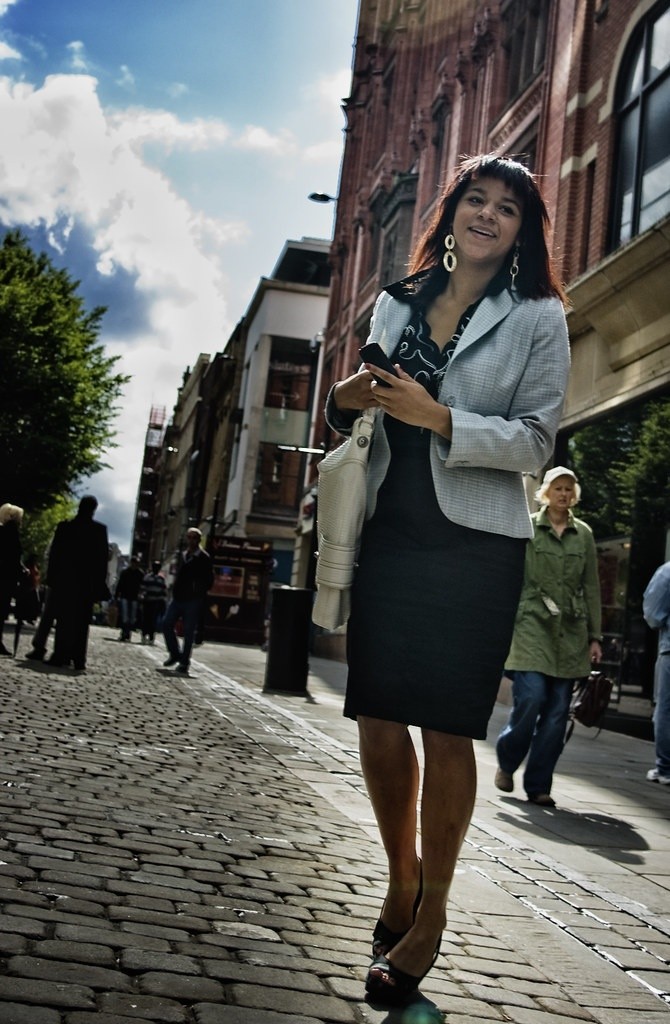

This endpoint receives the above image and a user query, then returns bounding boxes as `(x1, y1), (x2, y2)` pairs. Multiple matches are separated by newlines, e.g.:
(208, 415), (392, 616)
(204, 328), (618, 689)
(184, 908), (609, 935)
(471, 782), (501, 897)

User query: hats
(544, 466), (578, 483)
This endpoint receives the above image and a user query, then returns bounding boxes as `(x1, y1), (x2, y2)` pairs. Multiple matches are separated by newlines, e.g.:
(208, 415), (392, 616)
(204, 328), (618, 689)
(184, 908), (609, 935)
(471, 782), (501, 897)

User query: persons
(115, 527), (215, 673)
(0, 496), (111, 671)
(642, 561), (670, 785)
(495, 466), (605, 806)
(324, 155), (574, 997)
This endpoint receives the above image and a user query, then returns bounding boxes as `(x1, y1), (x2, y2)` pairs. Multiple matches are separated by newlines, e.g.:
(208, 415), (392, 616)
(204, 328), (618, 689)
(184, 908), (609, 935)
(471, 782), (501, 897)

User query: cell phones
(358, 341), (400, 389)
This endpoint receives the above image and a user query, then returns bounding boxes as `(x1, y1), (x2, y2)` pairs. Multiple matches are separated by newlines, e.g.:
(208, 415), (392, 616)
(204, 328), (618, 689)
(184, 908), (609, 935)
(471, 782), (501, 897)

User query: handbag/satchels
(567, 670), (614, 727)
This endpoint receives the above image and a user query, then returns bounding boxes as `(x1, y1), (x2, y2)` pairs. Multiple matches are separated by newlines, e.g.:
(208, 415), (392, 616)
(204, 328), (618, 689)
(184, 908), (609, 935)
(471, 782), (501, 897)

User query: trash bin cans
(264, 589), (314, 696)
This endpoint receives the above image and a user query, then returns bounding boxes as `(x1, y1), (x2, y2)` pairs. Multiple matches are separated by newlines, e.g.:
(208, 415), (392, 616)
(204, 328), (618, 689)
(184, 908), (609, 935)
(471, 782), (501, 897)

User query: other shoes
(163, 649), (184, 666)
(646, 769), (669, 784)
(42, 652), (65, 667)
(74, 660), (87, 670)
(494, 767), (514, 792)
(175, 663), (190, 672)
(25, 647), (47, 660)
(529, 793), (555, 808)
(0, 643), (12, 655)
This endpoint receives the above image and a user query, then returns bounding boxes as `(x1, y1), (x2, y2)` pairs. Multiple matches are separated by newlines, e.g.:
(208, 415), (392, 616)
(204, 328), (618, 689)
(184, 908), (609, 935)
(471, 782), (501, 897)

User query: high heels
(374, 858), (423, 957)
(364, 928), (442, 1001)
